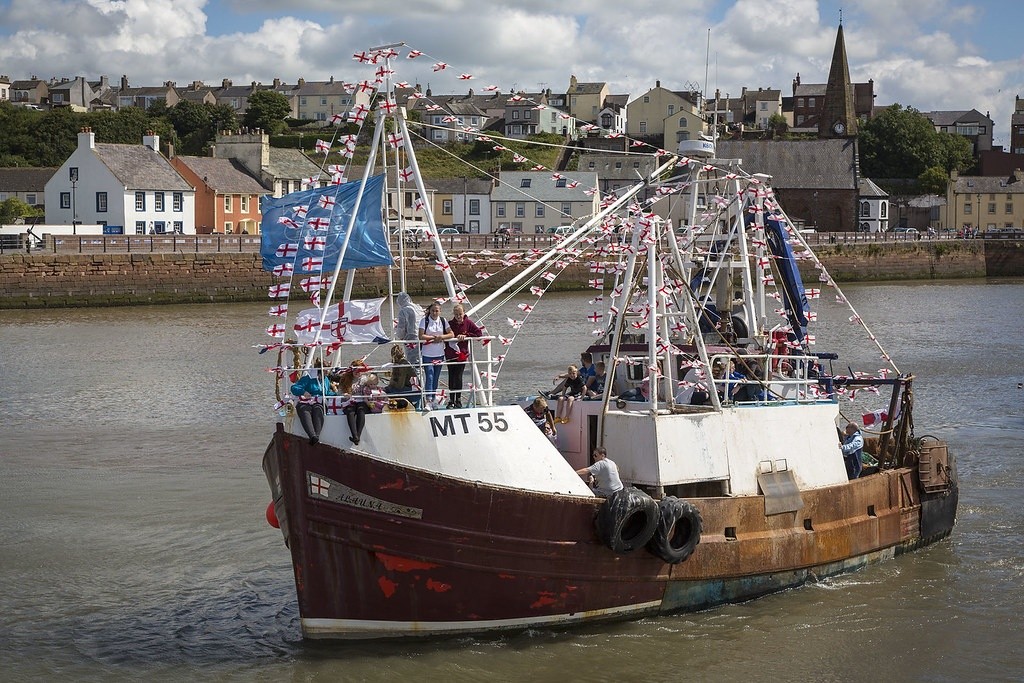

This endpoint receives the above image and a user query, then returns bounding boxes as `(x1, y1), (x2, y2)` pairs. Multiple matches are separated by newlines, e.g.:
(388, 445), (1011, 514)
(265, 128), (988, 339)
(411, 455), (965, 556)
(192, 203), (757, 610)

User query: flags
(738, 177), (901, 430)
(252, 48), (581, 415)
(582, 124), (737, 391)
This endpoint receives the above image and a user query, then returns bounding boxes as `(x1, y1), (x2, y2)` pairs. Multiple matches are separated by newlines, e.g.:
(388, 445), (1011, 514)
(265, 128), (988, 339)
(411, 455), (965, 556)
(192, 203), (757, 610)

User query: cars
(435, 227), (460, 235)
(894, 228), (919, 236)
(545, 226), (576, 234)
(675, 226), (706, 234)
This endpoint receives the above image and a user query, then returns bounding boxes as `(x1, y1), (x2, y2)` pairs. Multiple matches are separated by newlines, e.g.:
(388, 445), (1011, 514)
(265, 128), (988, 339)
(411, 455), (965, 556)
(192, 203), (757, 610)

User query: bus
(990, 228), (1022, 239)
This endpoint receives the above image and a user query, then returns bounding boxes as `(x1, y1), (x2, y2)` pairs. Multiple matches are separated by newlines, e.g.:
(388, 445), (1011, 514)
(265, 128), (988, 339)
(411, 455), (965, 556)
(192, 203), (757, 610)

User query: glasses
(593, 453), (600, 457)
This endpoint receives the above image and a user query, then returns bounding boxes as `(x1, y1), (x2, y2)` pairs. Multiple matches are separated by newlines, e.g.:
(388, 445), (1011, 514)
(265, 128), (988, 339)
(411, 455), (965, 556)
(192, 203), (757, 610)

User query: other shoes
(308, 436), (319, 445)
(433, 403), (438, 409)
(538, 390), (549, 398)
(456, 401), (462, 408)
(349, 436), (360, 445)
(446, 401), (454, 409)
(425, 403), (433, 412)
(583, 395), (590, 401)
(561, 417), (569, 424)
(548, 394), (553, 400)
(553, 417), (561, 424)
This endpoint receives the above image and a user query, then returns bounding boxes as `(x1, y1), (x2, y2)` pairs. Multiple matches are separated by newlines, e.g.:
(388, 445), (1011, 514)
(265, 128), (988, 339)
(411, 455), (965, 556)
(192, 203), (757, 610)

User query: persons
(523, 397), (557, 444)
(838, 422), (864, 480)
(538, 352), (615, 400)
(444, 305), (483, 409)
(575, 447), (624, 498)
(419, 303), (455, 412)
(364, 374), (387, 413)
(173, 228), (510, 248)
(290, 360), (331, 445)
(554, 365), (587, 424)
(382, 345), (421, 409)
(26, 238), (31, 254)
(338, 360), (366, 445)
(831, 225), (978, 243)
(673, 350), (763, 405)
(610, 360), (662, 402)
(393, 292), (423, 364)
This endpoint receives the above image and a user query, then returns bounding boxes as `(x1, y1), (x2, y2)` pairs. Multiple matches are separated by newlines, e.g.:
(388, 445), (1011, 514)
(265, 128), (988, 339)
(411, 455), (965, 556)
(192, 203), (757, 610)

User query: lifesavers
(646, 494), (704, 565)
(594, 486), (660, 555)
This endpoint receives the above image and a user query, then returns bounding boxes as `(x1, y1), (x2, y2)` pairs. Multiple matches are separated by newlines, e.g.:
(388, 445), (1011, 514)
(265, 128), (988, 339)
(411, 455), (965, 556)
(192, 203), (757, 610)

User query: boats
(260, 40), (959, 642)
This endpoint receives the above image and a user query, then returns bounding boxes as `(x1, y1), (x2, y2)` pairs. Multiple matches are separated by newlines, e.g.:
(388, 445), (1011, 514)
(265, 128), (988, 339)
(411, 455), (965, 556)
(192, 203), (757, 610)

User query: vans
(387, 220), (431, 237)
